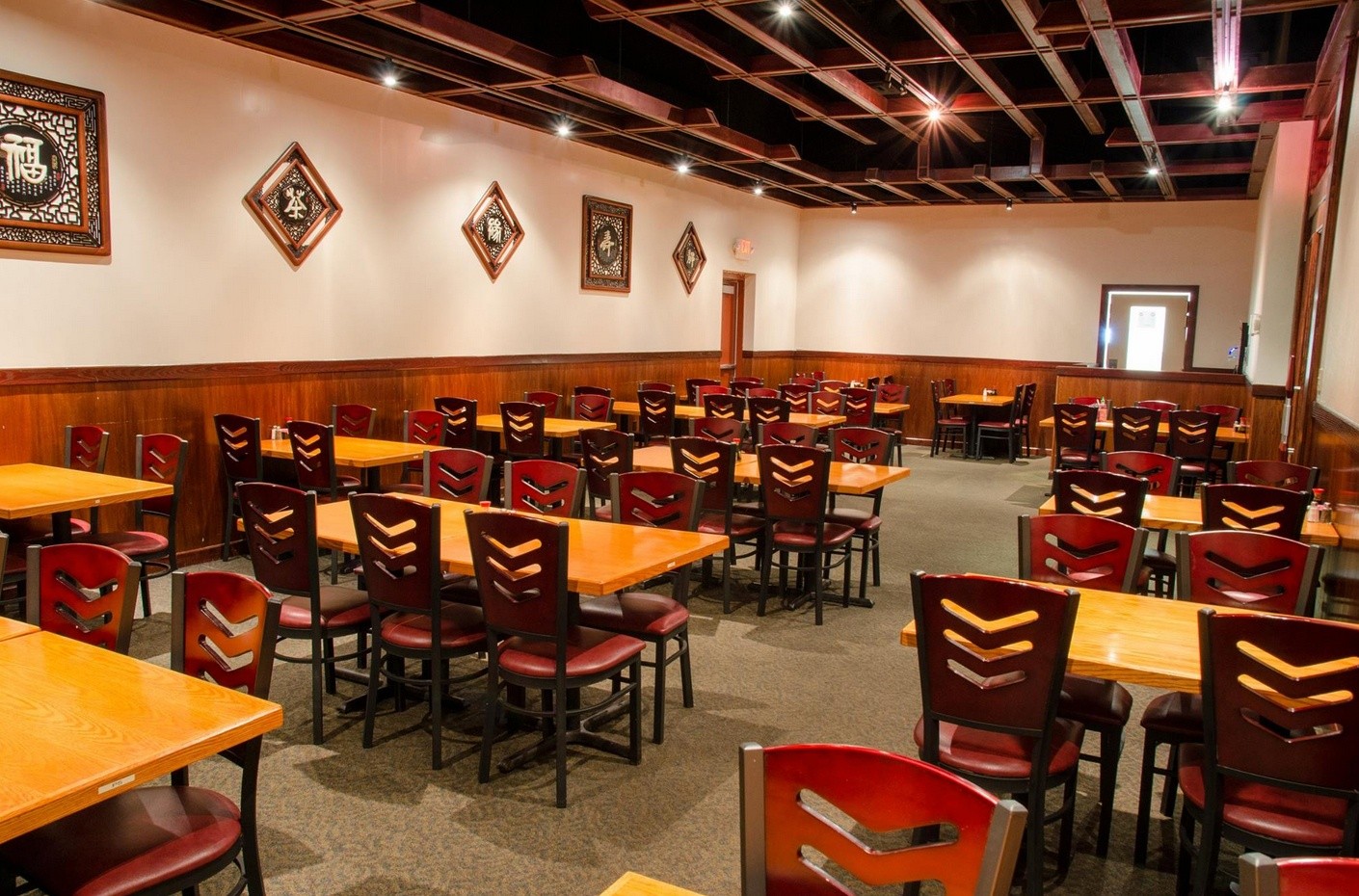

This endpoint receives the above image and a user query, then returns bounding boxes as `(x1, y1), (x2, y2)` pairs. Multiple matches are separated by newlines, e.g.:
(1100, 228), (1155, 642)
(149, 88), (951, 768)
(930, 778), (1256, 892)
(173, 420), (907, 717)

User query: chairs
(0, 371), (1359, 896)
